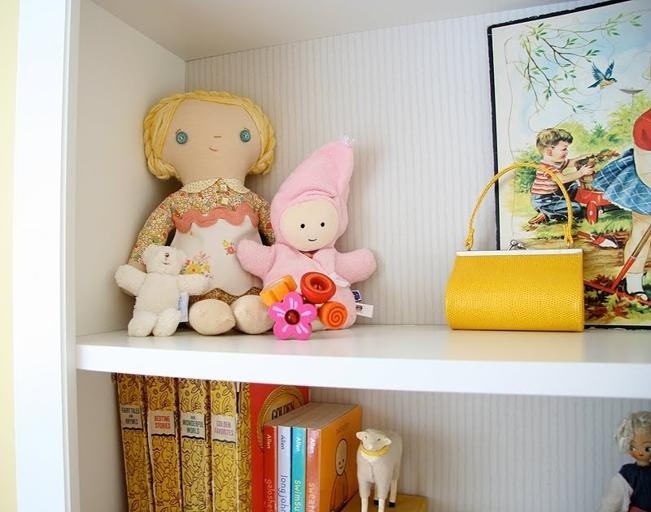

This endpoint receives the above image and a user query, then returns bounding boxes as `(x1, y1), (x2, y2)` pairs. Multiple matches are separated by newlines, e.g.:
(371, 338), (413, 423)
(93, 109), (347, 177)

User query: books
(113, 376), (363, 512)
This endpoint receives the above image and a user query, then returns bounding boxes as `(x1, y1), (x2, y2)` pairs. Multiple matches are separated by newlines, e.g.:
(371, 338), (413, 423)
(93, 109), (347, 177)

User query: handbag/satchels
(445, 163), (585, 332)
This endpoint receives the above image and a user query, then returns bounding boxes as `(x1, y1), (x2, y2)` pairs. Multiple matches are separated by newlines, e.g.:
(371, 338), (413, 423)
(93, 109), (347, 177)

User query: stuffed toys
(115, 244), (212, 338)
(355, 426), (403, 512)
(236, 136), (378, 333)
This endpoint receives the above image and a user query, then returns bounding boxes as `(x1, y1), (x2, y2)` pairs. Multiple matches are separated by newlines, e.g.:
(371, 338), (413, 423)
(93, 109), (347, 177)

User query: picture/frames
(487, 2), (650, 331)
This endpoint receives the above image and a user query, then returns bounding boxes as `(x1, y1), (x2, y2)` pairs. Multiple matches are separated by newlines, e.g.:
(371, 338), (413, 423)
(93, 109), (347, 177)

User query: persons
(121, 89), (277, 334)
(591, 108), (651, 303)
(600, 411), (651, 512)
(528, 127), (594, 225)
